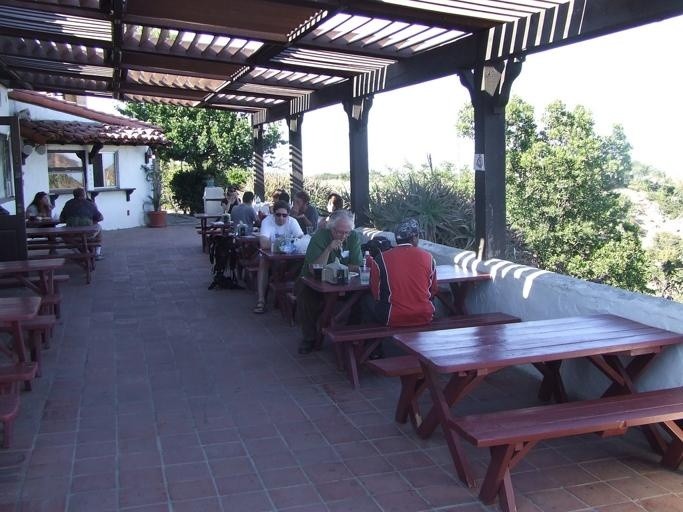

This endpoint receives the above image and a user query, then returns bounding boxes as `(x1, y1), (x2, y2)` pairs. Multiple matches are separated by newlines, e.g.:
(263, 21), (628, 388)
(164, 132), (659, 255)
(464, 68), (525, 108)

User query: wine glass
(285, 233), (292, 253)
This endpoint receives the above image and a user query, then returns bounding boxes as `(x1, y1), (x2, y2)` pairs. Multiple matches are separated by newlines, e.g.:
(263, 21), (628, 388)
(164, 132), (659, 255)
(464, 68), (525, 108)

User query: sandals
(252, 297), (266, 314)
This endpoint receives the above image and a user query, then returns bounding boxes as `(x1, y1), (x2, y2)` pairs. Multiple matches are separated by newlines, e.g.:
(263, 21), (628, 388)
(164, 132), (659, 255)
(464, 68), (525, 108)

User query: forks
(340, 247), (345, 259)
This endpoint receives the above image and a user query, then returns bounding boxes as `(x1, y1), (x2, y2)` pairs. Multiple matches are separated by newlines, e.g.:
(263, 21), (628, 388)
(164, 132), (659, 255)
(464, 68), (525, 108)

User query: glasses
(275, 211), (288, 217)
(229, 190), (236, 193)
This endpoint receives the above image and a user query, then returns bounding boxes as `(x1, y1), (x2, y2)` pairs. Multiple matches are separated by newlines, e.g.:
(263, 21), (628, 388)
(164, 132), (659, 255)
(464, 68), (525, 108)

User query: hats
(395, 217), (424, 239)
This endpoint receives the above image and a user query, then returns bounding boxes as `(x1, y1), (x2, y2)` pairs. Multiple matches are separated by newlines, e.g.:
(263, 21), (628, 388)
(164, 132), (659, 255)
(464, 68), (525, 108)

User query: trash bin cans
(201, 187), (225, 228)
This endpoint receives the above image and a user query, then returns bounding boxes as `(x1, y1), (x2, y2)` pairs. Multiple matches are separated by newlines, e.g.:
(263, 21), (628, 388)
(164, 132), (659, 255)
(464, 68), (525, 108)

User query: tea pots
(238, 225), (245, 236)
(271, 242), (280, 254)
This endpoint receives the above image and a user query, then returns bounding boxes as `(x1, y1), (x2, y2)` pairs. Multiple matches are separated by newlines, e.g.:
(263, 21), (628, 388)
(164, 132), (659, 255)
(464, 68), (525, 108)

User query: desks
(194, 212), (225, 250)
(391, 313), (682, 488)
(298, 264), (494, 374)
(0, 296), (43, 391)
(236, 234), (256, 286)
(26, 224), (101, 272)
(0, 258), (66, 319)
(256, 242), (305, 315)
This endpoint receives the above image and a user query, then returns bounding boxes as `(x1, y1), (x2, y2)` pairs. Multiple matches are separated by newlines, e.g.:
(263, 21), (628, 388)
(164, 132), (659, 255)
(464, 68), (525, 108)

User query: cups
(313, 263), (323, 281)
(359, 267), (371, 286)
(222, 214), (230, 227)
(304, 227), (313, 235)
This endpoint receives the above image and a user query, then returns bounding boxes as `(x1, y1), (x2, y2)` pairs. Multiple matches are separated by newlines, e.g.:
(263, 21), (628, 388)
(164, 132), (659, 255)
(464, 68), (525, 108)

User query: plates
(280, 245), (294, 251)
(349, 271), (358, 277)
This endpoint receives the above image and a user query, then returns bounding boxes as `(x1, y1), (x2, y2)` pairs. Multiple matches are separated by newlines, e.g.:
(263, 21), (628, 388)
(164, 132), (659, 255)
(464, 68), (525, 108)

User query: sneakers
(371, 346), (385, 358)
(299, 337), (313, 353)
(95, 255), (104, 260)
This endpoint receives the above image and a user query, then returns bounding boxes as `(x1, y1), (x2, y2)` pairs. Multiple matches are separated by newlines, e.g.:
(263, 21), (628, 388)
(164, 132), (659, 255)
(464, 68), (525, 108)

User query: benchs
(269, 279), (295, 319)
(2, 273), (71, 309)
(0, 361), (40, 390)
(25, 240), (104, 269)
(446, 385), (683, 512)
(0, 392), (23, 449)
(39, 293), (63, 337)
(321, 310), (521, 393)
(368, 355), (425, 434)
(27, 251), (95, 283)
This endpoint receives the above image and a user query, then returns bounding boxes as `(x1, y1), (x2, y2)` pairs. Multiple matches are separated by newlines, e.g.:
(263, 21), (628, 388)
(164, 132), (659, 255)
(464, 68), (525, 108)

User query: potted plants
(141, 157), (167, 229)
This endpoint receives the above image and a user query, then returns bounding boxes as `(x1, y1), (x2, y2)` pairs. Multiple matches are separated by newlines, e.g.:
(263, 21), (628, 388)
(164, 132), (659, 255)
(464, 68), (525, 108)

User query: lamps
(35, 143), (46, 155)
(21, 144), (33, 164)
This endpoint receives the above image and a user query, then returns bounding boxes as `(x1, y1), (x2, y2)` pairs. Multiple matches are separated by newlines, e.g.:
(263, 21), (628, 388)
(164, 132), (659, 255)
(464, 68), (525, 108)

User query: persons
(294, 208), (363, 354)
(220, 186), (241, 213)
(269, 192), (280, 214)
(280, 191), (291, 214)
(252, 200), (304, 313)
(230, 191), (261, 234)
(59, 188), (105, 261)
(317, 194), (343, 233)
(360, 219), (437, 326)
(26, 192), (59, 254)
(289, 192), (317, 234)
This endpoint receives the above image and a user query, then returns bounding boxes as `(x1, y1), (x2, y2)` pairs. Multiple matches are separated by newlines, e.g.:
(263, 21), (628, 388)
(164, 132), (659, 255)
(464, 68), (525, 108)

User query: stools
(0, 314), (57, 349)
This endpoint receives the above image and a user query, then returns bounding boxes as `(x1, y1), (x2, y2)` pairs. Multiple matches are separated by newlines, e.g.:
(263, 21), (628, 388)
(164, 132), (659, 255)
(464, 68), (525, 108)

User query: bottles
(237, 220), (245, 238)
(360, 250), (370, 284)
